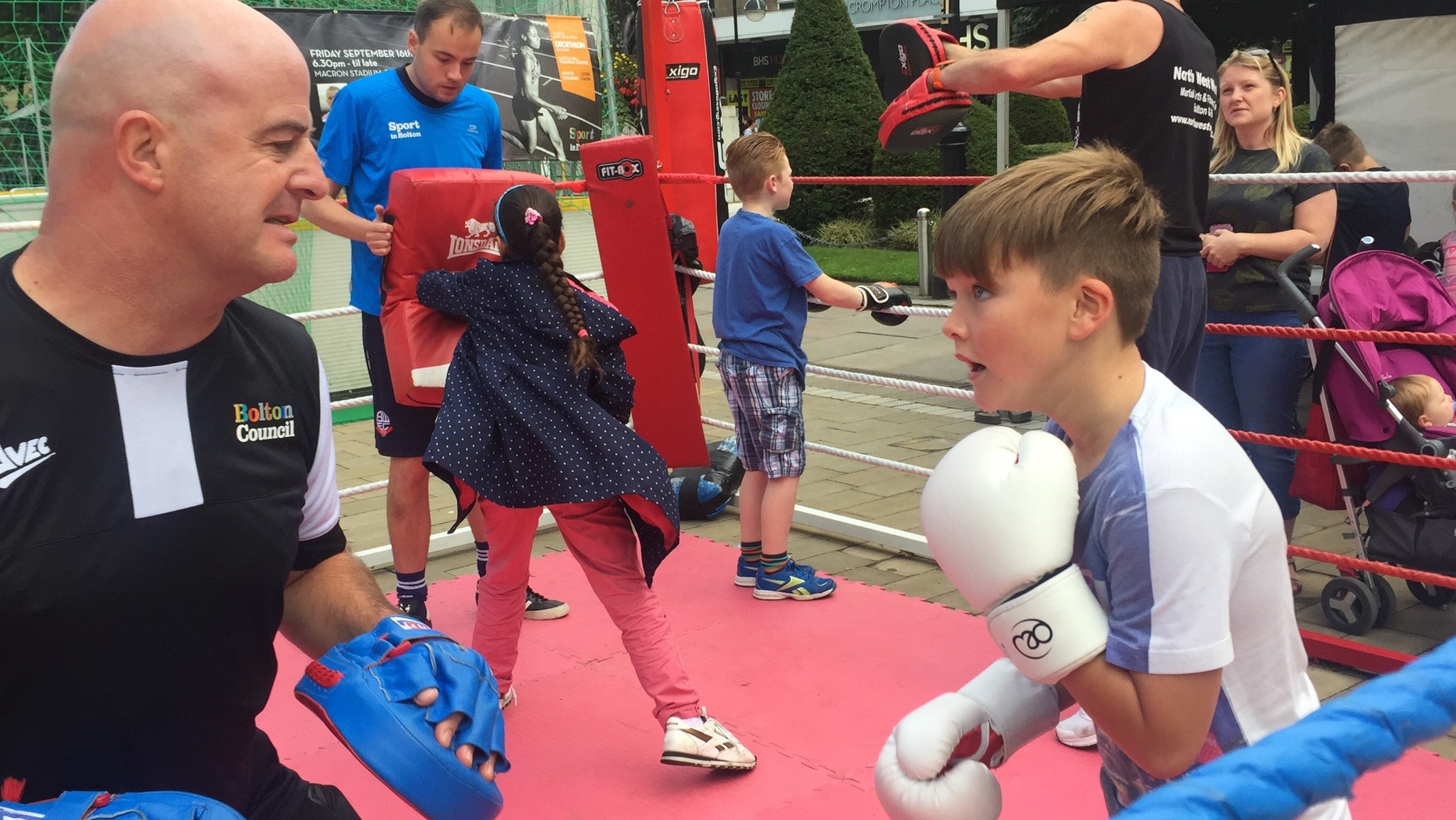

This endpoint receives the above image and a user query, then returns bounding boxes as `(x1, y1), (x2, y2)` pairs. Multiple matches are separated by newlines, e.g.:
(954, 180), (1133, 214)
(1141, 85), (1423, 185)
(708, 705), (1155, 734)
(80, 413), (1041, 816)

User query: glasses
(1236, 46), (1282, 86)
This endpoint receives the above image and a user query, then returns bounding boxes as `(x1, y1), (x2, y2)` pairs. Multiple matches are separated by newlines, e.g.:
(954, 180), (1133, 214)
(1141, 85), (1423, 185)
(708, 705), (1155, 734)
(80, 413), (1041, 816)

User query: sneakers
(658, 712), (759, 774)
(1058, 705), (1098, 749)
(751, 561), (836, 601)
(395, 600), (431, 629)
(471, 580), (571, 620)
(733, 555), (815, 585)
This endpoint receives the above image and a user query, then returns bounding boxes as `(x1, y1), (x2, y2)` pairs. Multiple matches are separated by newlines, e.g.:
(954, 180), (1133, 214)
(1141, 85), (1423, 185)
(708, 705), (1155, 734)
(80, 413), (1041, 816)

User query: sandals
(1285, 559), (1302, 596)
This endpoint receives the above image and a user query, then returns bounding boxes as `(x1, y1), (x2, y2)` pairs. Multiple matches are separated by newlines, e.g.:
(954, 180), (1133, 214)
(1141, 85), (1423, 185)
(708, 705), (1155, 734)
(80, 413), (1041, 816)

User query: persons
(1433, 180), (1456, 315)
(872, 0), (1223, 407)
(411, 181), (759, 778)
(1181, 46), (1342, 596)
(1, 0), (513, 820)
(295, 0), (571, 635)
(876, 137), (1362, 820)
(712, 135), (914, 602)
(1381, 375), (1456, 495)
(1310, 120), (1411, 370)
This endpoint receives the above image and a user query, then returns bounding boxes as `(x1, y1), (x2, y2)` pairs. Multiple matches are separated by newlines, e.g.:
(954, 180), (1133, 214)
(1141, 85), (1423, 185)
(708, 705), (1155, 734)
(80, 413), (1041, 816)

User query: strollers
(1274, 235), (1455, 639)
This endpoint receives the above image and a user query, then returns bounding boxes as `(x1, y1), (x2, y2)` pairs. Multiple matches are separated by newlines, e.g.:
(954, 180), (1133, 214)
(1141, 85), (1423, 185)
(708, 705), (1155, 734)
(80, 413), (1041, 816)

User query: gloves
(854, 281), (912, 326)
(806, 287), (833, 313)
(876, 664), (1065, 820)
(919, 427), (1107, 685)
